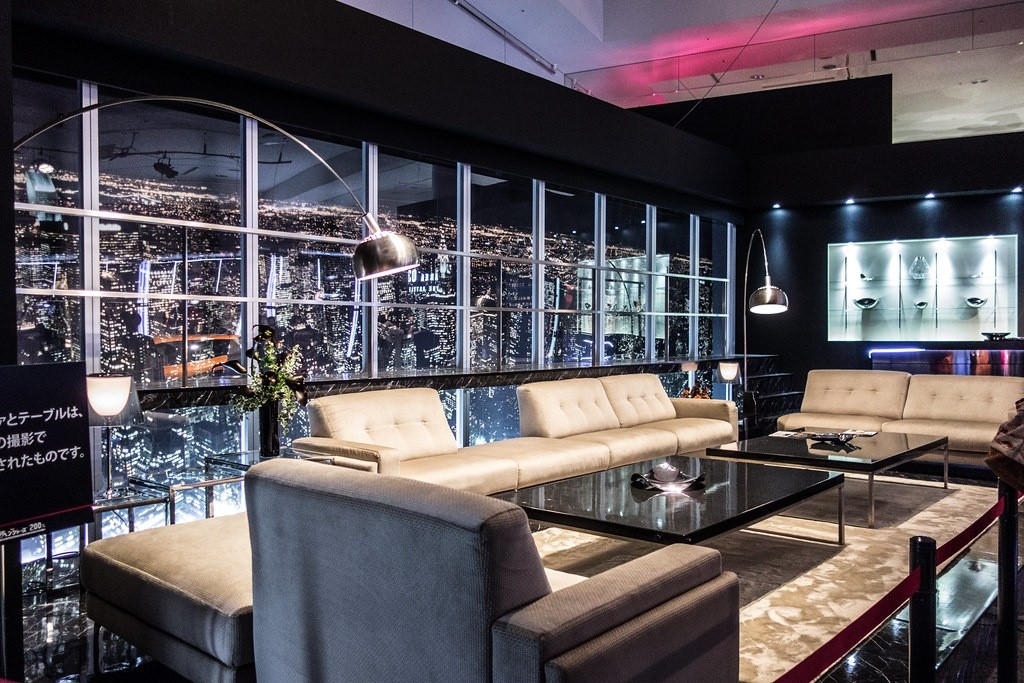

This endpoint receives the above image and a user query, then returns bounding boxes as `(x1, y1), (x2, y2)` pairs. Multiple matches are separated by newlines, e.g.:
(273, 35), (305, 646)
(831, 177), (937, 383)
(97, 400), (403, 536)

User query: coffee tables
(484, 451), (847, 591)
(706, 425), (949, 529)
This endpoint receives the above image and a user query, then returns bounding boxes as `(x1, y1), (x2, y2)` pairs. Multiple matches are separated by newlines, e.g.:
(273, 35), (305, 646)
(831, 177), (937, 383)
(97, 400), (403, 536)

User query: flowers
(680, 384), (711, 399)
(235, 322), (311, 435)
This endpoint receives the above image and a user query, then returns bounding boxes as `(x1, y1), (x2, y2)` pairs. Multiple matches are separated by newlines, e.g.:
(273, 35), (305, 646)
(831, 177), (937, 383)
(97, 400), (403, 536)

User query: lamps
(712, 360), (742, 400)
(12, 95), (422, 281)
(741, 227), (791, 391)
(85, 372), (144, 502)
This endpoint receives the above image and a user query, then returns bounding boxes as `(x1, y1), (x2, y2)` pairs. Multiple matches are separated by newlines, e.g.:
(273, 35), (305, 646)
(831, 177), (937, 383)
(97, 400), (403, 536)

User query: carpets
(539, 536), (847, 610)
(779, 475), (960, 530)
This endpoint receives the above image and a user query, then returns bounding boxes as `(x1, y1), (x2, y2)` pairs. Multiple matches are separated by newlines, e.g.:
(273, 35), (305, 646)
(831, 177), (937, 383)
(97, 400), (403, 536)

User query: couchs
(79, 449), (740, 682)
(777, 368), (1024, 453)
(302, 368), (742, 522)
